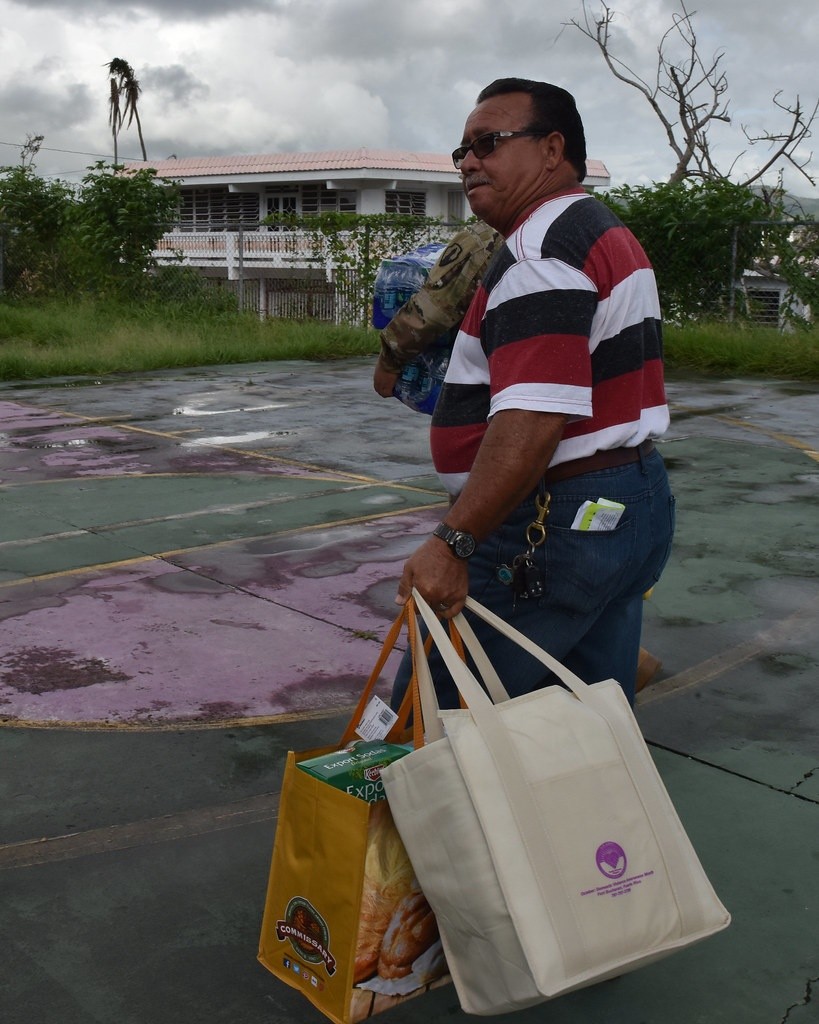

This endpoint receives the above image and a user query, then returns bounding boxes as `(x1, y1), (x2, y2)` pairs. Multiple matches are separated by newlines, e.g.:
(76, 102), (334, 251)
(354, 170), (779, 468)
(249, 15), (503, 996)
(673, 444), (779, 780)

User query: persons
(391, 77), (676, 733)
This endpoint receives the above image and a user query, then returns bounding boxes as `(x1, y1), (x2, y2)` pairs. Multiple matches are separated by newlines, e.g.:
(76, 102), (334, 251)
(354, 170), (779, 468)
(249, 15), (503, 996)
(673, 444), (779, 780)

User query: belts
(536, 439), (656, 486)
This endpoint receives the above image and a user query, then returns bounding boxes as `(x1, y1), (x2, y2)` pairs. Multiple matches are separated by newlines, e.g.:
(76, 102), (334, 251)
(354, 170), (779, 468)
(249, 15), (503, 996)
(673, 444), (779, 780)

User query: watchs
(433, 520), (480, 564)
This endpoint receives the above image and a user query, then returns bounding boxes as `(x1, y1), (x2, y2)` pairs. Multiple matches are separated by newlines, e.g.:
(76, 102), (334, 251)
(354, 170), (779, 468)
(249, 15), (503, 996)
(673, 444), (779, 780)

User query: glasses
(452, 130), (548, 169)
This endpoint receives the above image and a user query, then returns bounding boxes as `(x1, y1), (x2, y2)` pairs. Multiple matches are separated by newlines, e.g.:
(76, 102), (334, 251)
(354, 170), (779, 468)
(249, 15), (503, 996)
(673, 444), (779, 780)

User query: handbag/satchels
(255, 596), (468, 1024)
(380, 587), (731, 1015)
